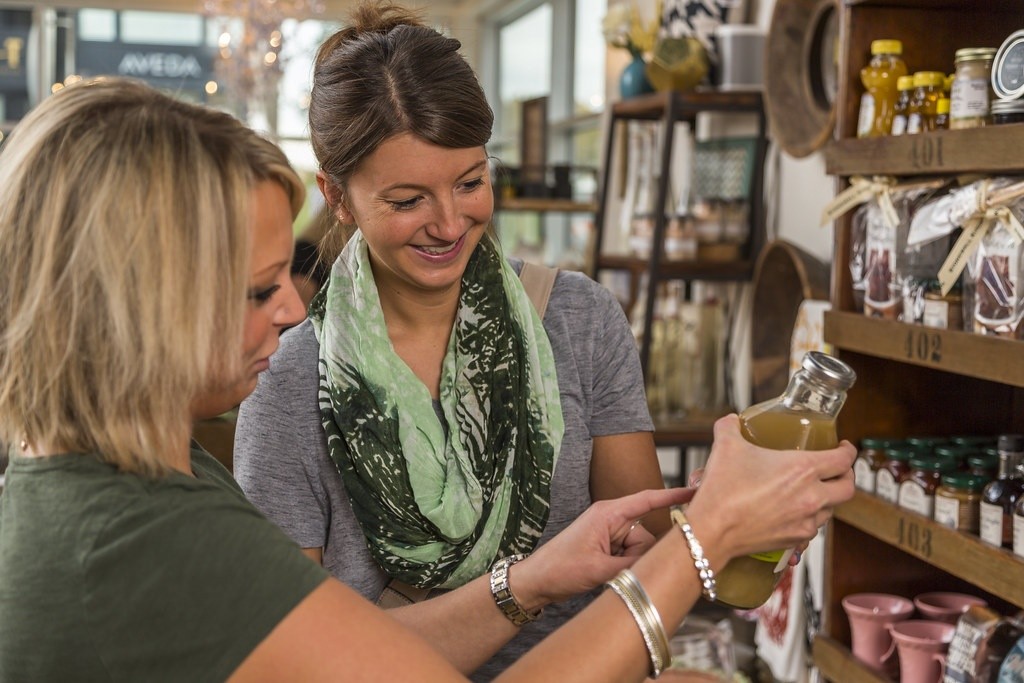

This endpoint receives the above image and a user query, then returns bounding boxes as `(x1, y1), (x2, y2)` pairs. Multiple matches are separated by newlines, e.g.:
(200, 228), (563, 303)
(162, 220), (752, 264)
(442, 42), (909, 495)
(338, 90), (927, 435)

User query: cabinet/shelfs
(482, 0), (1024, 683)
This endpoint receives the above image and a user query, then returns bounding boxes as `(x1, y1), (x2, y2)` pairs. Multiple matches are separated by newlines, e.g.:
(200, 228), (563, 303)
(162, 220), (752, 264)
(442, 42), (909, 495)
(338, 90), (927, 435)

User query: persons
(0, 79), (858, 683)
(232, 4), (672, 683)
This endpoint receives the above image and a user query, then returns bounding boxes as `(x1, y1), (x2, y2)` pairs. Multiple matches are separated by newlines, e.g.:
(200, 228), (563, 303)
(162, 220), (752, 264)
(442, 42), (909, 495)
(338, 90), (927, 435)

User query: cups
(841, 590), (1024, 683)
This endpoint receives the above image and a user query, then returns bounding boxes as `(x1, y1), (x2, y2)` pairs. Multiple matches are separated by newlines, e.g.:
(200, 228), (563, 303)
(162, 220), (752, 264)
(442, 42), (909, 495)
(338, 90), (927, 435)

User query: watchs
(491, 553), (543, 625)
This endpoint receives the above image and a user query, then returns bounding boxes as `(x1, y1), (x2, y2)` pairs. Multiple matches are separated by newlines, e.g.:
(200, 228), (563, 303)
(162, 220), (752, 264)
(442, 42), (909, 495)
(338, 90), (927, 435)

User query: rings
(629, 519), (641, 531)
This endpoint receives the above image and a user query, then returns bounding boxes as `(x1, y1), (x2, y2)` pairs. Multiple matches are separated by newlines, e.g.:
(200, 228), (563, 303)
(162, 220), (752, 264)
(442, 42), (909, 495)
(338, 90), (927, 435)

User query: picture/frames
(521, 92), (548, 184)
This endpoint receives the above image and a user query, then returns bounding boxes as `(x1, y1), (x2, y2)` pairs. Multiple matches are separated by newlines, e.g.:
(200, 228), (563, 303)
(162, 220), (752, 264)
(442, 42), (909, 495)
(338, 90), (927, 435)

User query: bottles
(851, 425), (1024, 568)
(667, 351), (857, 611)
(597, 273), (729, 418)
(854, 35), (1024, 136)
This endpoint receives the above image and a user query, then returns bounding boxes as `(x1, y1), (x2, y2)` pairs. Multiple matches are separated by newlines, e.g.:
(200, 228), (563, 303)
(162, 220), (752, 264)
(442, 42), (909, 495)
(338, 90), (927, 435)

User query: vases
(619, 56), (651, 98)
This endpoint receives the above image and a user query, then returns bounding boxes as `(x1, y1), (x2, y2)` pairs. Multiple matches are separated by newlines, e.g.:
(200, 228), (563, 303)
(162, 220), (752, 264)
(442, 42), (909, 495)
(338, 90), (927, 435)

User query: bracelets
(671, 509), (718, 601)
(605, 569), (672, 679)
